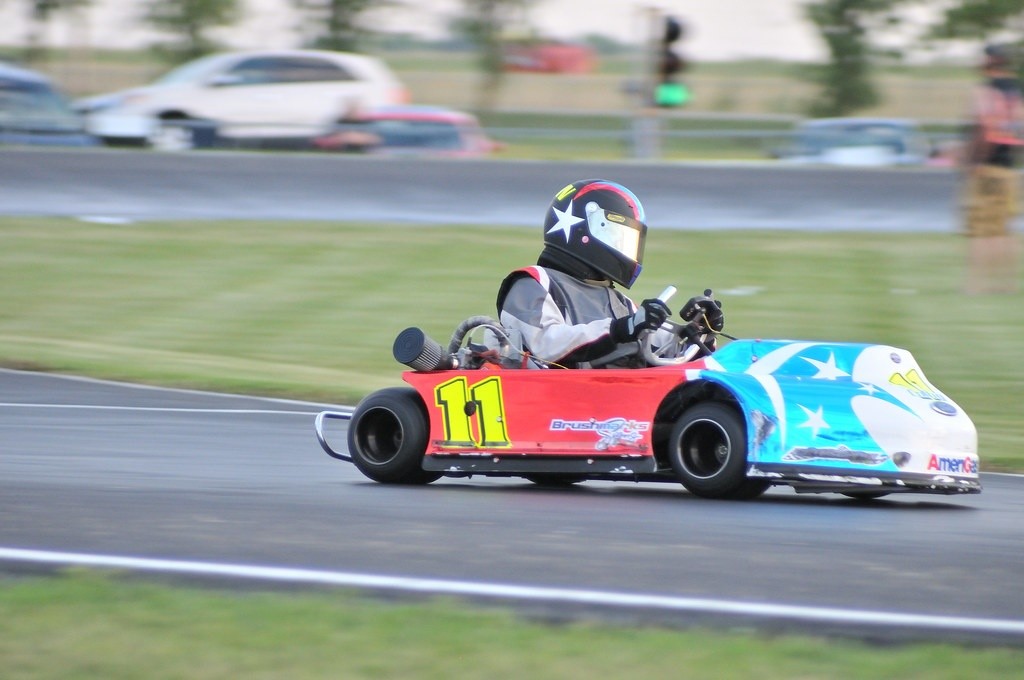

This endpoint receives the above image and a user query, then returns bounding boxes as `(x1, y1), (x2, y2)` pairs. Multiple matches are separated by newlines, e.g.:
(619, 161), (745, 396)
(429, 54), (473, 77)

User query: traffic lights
(647, 15), (688, 106)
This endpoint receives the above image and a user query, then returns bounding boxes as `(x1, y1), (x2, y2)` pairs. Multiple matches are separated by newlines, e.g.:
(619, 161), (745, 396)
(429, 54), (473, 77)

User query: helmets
(544, 178), (648, 290)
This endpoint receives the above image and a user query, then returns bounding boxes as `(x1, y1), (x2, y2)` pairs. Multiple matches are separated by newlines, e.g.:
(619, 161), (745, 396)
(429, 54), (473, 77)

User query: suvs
(79, 45), (407, 151)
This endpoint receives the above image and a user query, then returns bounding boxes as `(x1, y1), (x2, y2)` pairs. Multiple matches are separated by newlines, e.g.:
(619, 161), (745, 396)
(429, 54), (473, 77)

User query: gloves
(618, 298), (672, 344)
(679, 296), (724, 334)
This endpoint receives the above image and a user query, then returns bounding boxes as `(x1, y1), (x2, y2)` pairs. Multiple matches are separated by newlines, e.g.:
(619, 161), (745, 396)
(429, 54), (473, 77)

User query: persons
(496, 178), (724, 370)
(954, 36), (1024, 171)
(628, 18), (694, 124)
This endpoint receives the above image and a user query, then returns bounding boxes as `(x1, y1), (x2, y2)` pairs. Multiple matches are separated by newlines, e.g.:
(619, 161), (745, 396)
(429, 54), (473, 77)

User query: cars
(779, 119), (930, 168)
